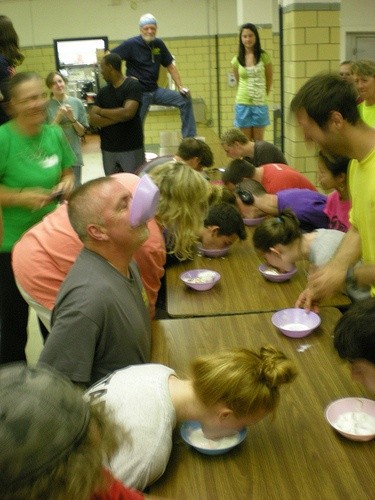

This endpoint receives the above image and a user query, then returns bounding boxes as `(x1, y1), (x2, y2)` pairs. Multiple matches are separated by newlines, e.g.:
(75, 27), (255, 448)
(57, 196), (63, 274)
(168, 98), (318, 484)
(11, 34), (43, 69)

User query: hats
(138, 13), (157, 28)
(0, 362), (91, 488)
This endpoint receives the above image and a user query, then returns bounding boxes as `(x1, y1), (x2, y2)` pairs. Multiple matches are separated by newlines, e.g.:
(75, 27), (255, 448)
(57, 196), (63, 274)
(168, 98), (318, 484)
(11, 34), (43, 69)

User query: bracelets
(70, 120), (77, 123)
(98, 108), (102, 114)
(345, 263), (358, 290)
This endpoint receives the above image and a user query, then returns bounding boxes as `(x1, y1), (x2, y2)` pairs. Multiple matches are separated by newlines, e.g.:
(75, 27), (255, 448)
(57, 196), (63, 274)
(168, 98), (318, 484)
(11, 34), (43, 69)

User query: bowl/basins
(198, 244), (230, 258)
(271, 308), (322, 337)
(325, 397), (375, 441)
(181, 420), (248, 455)
(259, 262), (299, 282)
(180, 270), (220, 290)
(242, 216), (267, 225)
(131, 175), (160, 228)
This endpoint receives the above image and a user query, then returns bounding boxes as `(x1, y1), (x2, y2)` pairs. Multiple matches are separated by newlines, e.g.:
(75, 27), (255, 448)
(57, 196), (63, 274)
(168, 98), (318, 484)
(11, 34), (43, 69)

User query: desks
(158, 144), (355, 316)
(160, 125), (221, 147)
(149, 306), (375, 500)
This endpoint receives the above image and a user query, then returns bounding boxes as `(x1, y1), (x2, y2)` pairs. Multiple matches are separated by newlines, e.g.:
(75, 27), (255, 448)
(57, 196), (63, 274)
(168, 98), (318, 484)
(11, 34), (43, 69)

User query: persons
(37, 179), (152, 390)
(334, 298), (375, 399)
(232, 24), (272, 142)
(109, 14), (196, 138)
(339, 60), (375, 128)
(0, 15), (24, 126)
(87, 54), (148, 176)
(45, 72), (89, 190)
(0, 344), (299, 500)
(11, 155), (212, 335)
(139, 129), (370, 300)
(0, 73), (82, 367)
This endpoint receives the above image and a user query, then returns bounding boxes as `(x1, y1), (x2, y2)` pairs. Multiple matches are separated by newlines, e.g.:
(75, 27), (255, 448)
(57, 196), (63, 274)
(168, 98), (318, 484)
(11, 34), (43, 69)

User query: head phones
(235, 181), (254, 205)
